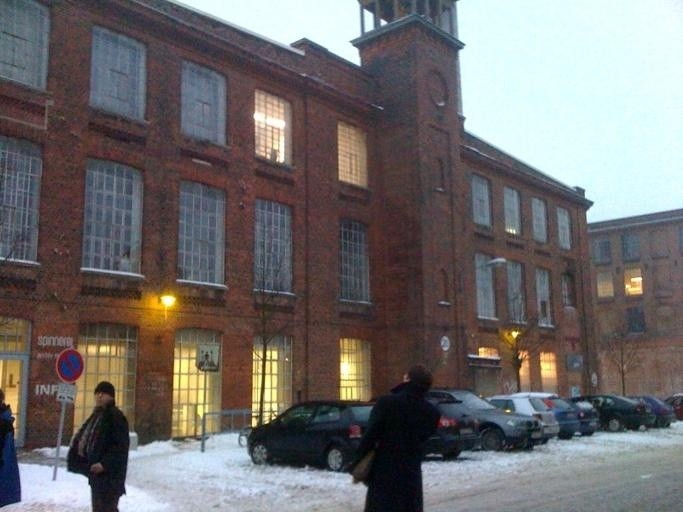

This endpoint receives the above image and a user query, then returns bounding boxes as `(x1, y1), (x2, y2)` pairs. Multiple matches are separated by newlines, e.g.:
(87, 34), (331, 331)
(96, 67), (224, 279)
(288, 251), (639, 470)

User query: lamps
(459, 258), (508, 291)
(160, 271), (177, 307)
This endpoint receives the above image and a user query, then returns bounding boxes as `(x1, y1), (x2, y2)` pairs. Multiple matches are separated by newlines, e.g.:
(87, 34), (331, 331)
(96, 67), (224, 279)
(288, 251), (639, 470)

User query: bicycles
(237, 414), (275, 448)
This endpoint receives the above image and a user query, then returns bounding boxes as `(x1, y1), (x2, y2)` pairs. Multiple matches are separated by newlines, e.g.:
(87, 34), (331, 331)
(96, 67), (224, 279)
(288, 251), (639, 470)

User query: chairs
(319, 410), (330, 422)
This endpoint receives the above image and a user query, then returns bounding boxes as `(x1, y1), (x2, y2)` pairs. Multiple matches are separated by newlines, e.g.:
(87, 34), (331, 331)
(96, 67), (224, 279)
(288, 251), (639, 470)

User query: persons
(67, 379), (130, 511)
(0, 387), (20, 508)
(349, 364), (441, 511)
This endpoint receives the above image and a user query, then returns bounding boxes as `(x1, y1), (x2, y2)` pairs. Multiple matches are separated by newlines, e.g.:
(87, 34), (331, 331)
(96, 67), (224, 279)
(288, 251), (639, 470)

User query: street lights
(460, 257), (509, 390)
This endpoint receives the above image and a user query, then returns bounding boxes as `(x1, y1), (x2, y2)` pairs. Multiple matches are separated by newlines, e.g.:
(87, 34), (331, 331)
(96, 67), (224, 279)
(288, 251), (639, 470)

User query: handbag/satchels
(352, 448), (376, 484)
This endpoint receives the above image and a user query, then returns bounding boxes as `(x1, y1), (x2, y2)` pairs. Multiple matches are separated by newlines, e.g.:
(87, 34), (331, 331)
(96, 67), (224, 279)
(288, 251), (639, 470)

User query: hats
(93, 381), (115, 397)
